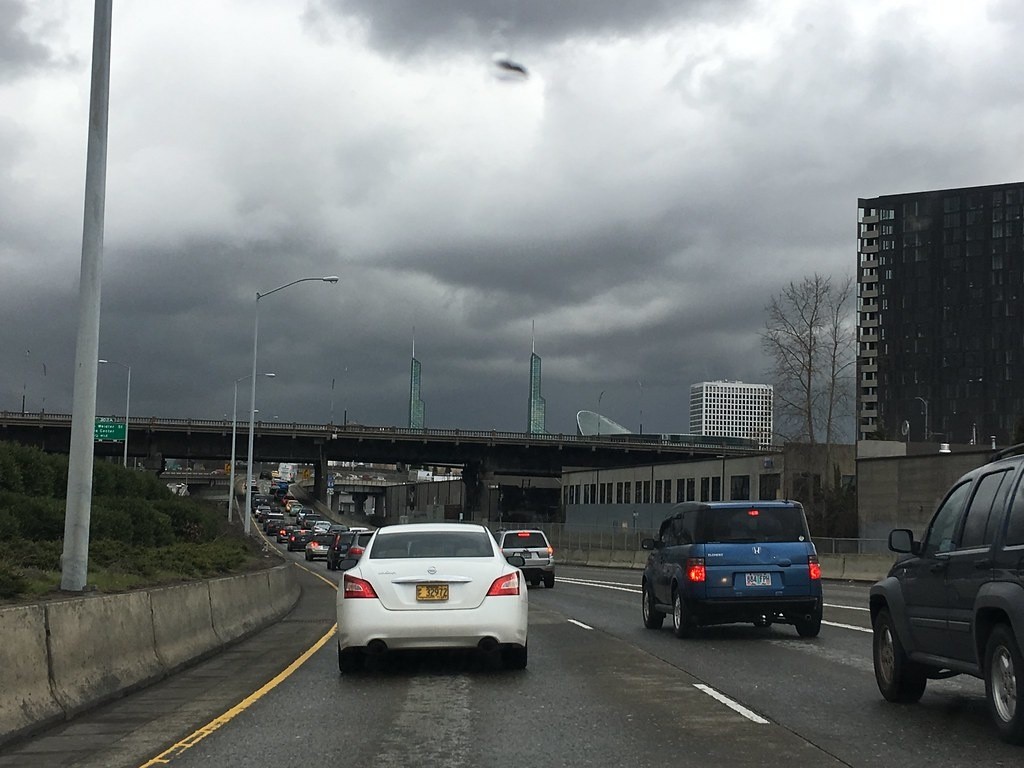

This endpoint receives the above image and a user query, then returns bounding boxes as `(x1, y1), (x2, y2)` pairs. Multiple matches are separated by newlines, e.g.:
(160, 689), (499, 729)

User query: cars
(327, 531), (375, 571)
(296, 509), (315, 525)
(266, 520), (285, 536)
(244, 476), (257, 491)
(287, 530), (313, 552)
(305, 533), (335, 561)
(311, 521), (369, 533)
(259, 471), (269, 479)
(281, 496), (295, 507)
(277, 523), (299, 543)
(263, 511), (284, 532)
(301, 514), (321, 529)
(641, 500), (823, 638)
(290, 504), (304, 516)
(268, 471), (288, 494)
(250, 491), (272, 523)
(286, 500), (298, 512)
(336, 523), (528, 674)
(274, 489), (288, 501)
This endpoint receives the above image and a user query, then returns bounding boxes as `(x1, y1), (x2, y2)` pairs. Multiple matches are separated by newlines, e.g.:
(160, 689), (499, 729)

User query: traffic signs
(94, 418), (126, 440)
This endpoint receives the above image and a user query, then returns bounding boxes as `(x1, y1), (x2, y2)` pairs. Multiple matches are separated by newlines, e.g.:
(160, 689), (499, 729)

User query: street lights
(98, 359), (131, 468)
(245, 276), (339, 536)
(228, 372), (276, 522)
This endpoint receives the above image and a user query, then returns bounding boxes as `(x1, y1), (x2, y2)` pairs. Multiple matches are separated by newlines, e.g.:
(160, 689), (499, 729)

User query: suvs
(870, 443), (1024, 746)
(493, 527), (555, 588)
